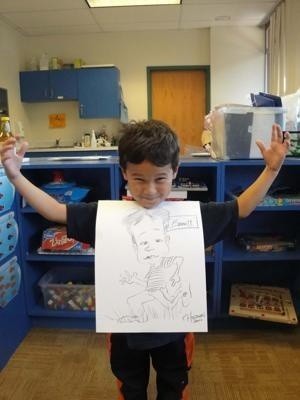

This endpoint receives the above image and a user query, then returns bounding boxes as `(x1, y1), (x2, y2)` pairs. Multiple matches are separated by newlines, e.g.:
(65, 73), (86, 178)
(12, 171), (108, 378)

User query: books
(237, 232), (294, 253)
(229, 281), (298, 325)
(229, 189), (300, 206)
(121, 177), (209, 201)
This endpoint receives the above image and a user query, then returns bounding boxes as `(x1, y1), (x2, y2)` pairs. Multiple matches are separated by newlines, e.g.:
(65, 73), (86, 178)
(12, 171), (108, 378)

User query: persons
(0, 119), (292, 399)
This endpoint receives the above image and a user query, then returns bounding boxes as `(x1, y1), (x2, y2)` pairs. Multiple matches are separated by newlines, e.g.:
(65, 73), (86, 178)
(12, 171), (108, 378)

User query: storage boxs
(211, 106), (289, 159)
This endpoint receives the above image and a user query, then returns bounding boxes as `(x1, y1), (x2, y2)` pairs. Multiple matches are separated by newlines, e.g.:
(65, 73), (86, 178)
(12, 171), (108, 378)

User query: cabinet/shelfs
(20, 69), (78, 103)
(77, 69), (122, 119)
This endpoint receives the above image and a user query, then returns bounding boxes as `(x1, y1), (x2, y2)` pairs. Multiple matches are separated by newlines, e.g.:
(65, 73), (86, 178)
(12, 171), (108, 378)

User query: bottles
(0, 116), (16, 156)
(91, 130), (97, 148)
(84, 133), (90, 148)
(31, 49), (88, 71)
